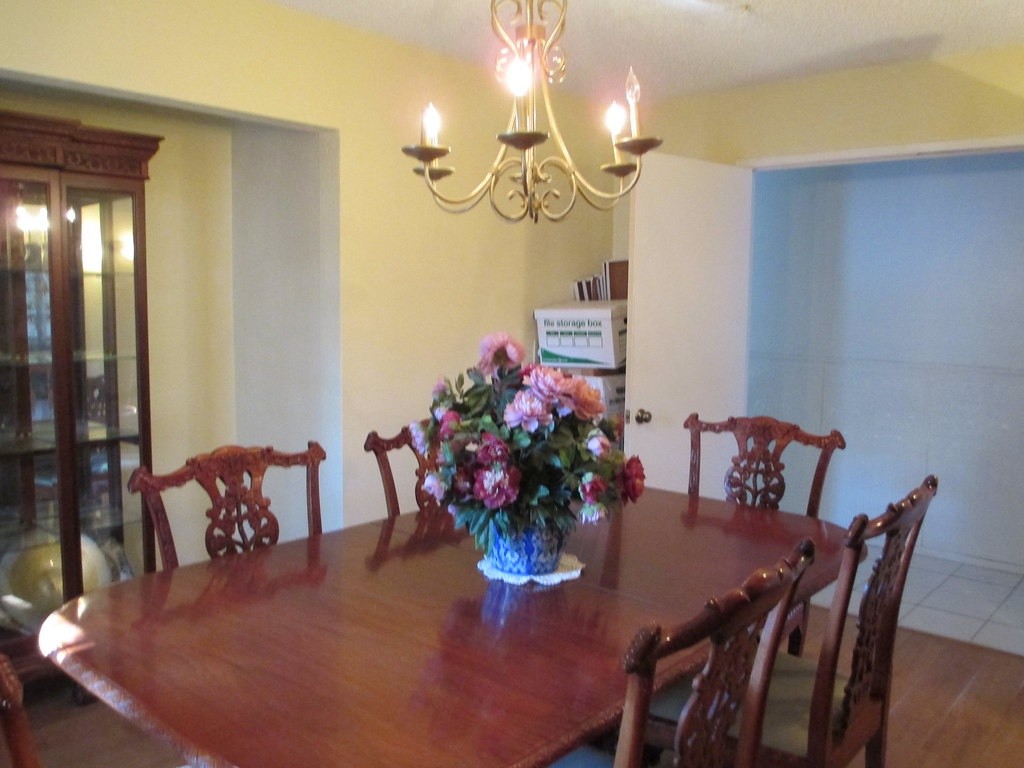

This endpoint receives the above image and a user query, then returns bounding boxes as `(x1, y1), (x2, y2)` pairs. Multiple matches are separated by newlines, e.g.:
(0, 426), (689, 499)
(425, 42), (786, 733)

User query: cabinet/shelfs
(1, 111), (163, 695)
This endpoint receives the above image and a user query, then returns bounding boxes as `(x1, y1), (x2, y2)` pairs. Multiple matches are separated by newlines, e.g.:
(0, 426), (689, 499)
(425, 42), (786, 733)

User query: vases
(484, 518), (563, 574)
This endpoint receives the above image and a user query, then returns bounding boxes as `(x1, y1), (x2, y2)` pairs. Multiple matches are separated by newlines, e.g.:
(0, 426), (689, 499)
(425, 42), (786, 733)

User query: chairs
(685, 413), (845, 517)
(724, 476), (938, 768)
(553, 540), (816, 768)
(364, 416), (445, 517)
(128, 440), (325, 570)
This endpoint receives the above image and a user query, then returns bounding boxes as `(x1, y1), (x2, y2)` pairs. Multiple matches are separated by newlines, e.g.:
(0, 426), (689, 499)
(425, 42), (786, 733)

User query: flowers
(411, 332), (644, 556)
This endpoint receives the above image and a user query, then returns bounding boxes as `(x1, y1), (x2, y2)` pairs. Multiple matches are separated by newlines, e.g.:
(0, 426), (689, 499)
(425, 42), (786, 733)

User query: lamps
(400, 0), (661, 222)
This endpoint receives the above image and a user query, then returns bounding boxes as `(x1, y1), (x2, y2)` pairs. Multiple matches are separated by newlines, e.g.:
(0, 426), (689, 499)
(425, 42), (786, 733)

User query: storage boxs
(534, 301), (627, 369)
(578, 375), (625, 422)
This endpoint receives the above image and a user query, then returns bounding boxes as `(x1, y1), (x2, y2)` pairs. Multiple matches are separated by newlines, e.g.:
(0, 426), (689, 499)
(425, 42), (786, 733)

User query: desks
(40, 487), (866, 768)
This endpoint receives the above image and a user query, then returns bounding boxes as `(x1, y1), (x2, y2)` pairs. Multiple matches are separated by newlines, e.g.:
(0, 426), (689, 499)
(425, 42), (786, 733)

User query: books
(576, 259), (628, 300)
